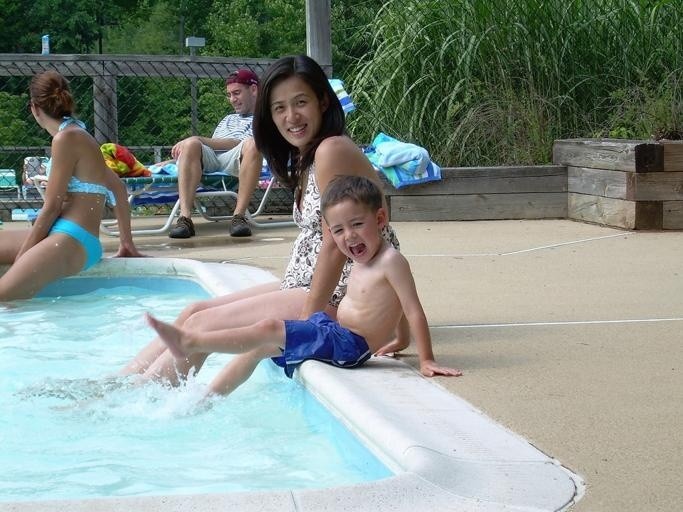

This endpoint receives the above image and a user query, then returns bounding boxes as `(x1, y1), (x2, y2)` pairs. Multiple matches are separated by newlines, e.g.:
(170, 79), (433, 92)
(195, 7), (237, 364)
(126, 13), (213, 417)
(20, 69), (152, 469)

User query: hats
(227, 69), (260, 87)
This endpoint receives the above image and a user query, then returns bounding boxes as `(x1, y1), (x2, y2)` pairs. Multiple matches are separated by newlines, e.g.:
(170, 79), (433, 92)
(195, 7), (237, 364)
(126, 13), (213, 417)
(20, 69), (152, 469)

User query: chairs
(22, 157), (50, 199)
(33, 79), (354, 238)
(0, 168), (20, 201)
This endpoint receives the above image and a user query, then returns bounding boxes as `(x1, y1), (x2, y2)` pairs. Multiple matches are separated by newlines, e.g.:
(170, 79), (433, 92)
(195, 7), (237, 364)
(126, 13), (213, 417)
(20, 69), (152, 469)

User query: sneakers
(231, 214), (252, 236)
(169, 217), (196, 238)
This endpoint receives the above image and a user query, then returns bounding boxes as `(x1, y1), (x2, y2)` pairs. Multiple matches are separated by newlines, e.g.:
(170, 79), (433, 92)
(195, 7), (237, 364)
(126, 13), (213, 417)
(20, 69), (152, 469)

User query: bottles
(41, 34), (49, 56)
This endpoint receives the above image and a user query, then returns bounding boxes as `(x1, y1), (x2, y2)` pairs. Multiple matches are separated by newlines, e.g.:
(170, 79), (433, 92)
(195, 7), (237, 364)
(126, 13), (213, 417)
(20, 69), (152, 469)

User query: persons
(166, 69), (268, 239)
(138, 174), (463, 413)
(113, 51), (389, 388)
(20, 162), (48, 186)
(1, 68), (147, 306)
